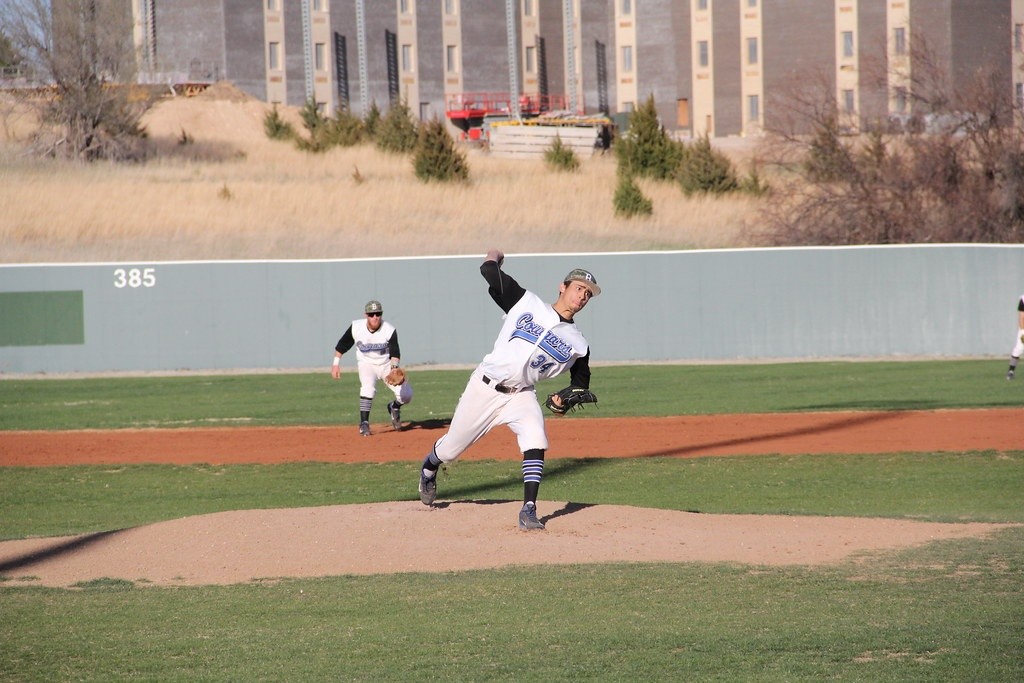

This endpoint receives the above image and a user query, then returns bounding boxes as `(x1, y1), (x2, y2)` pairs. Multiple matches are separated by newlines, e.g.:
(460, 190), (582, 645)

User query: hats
(365, 301), (382, 313)
(564, 268), (602, 298)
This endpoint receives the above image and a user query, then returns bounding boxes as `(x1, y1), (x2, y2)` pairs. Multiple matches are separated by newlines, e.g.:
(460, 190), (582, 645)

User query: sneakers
(360, 422), (371, 437)
(388, 401), (402, 431)
(519, 504), (545, 532)
(418, 456), (439, 505)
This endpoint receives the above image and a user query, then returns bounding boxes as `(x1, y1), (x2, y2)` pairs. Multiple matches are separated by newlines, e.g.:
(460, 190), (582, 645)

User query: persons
(332, 300), (413, 437)
(418, 248), (602, 529)
(1006, 295), (1024, 380)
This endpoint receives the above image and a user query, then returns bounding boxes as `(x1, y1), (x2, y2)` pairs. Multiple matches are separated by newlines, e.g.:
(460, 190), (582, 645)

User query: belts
(483, 375), (518, 393)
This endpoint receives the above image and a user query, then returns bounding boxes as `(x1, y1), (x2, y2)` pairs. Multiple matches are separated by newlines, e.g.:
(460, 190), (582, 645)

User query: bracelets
(333, 357), (339, 365)
(391, 362), (399, 368)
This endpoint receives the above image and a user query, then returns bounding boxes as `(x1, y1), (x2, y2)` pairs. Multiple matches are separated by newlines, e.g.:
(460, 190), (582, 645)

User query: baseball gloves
(542, 385), (599, 417)
(385, 365), (405, 387)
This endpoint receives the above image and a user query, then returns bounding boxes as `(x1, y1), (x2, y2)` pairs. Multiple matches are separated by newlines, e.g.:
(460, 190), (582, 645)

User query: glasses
(367, 313), (382, 317)
